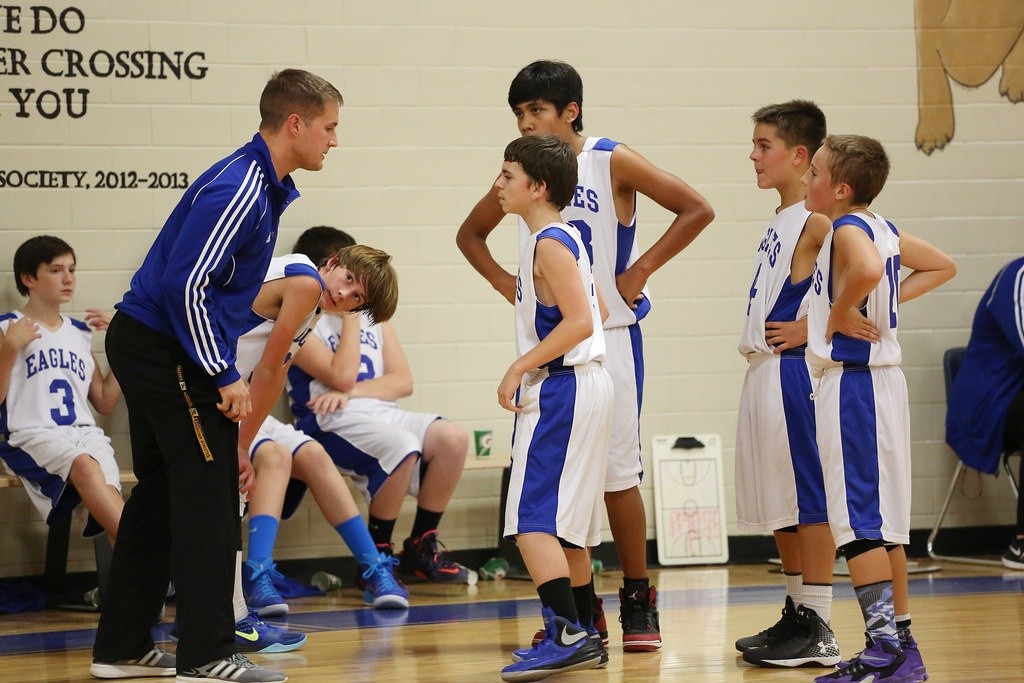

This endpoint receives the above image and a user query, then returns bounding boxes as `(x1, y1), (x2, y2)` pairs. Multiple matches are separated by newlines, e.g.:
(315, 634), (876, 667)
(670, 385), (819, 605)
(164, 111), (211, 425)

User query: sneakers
(512, 609), (610, 670)
(397, 528), (469, 583)
(501, 607), (602, 682)
(836, 619), (928, 683)
(241, 562), (288, 617)
(532, 599), (609, 651)
(735, 595), (796, 654)
(362, 551), (409, 609)
(232, 611), (307, 654)
(814, 633), (921, 683)
(176, 654), (288, 683)
(355, 544), (411, 596)
(1001, 538), (1024, 571)
(618, 579), (662, 651)
(740, 604), (842, 669)
(90, 644), (180, 679)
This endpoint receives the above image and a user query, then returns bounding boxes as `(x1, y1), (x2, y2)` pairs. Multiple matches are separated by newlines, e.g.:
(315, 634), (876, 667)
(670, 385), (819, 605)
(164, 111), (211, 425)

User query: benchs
(0, 457), (516, 604)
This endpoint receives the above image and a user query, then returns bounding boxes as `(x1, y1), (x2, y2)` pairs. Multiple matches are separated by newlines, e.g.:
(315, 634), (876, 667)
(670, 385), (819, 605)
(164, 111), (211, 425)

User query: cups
(591, 559), (602, 574)
(450, 563), (479, 585)
(474, 429), (493, 458)
(311, 571), (342, 593)
(480, 557), (510, 580)
(84, 586), (99, 607)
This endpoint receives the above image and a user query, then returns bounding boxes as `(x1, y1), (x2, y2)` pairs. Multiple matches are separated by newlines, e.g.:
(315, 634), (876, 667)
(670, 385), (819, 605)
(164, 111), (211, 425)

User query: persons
(455, 60), (718, 681)
(734, 100), (958, 683)
(946, 254), (1024, 569)
(0, 236), (129, 610)
(169, 225), (476, 652)
(89, 68), (345, 683)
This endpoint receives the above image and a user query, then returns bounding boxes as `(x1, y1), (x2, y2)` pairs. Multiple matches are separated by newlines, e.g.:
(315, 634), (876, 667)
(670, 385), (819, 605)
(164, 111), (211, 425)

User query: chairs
(927, 348), (1024, 567)
